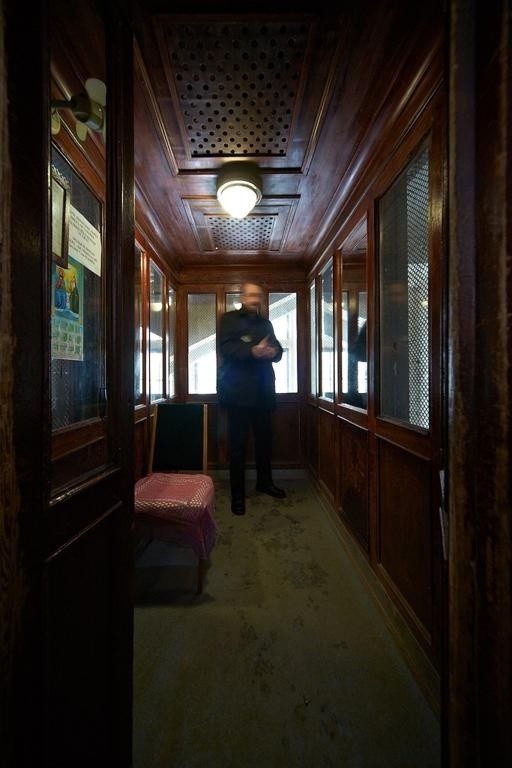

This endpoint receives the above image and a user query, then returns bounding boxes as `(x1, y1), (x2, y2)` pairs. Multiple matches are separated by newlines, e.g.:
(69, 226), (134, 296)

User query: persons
(216, 281), (286, 516)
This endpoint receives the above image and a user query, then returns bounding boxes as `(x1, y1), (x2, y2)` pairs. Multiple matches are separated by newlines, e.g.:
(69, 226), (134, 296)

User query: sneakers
(255, 483), (287, 498)
(231, 494), (246, 515)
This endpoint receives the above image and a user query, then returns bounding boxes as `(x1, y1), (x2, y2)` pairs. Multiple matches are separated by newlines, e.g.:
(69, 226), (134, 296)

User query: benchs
(134, 473), (219, 596)
